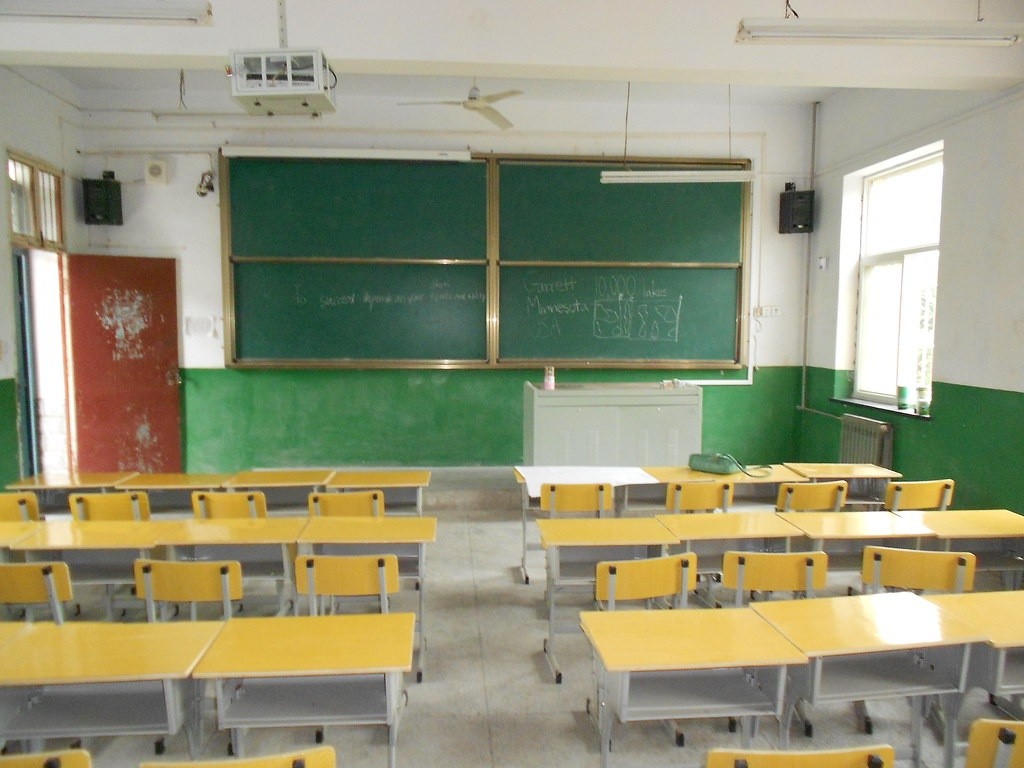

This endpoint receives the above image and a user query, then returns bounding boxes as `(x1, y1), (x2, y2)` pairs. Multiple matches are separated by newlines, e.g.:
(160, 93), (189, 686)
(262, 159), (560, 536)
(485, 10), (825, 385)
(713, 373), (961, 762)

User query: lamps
(600, 82), (754, 184)
(0, 0), (214, 26)
(735, 0), (1024, 47)
(151, 69), (320, 123)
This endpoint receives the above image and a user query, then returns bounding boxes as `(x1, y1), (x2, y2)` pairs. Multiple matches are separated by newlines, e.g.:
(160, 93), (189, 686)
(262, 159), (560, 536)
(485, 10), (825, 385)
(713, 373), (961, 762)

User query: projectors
(231, 48), (337, 118)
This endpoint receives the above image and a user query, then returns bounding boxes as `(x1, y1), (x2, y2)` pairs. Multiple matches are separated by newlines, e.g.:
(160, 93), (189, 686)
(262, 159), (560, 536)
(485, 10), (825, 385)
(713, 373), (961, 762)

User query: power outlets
(762, 307), (772, 317)
(753, 306), (762, 317)
(772, 306), (781, 316)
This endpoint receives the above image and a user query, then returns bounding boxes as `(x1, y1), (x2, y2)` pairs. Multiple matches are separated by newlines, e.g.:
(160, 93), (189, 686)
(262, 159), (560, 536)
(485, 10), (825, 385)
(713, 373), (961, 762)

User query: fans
(396, 75), (526, 132)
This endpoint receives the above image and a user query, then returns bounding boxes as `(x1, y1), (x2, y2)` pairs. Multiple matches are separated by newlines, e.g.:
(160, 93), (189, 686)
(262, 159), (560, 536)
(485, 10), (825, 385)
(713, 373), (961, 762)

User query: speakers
(81, 178), (124, 227)
(146, 160), (166, 185)
(779, 190), (815, 234)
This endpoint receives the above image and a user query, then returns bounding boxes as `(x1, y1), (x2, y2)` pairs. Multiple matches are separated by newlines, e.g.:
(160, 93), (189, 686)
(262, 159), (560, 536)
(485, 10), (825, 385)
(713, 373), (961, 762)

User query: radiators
(839, 413), (895, 505)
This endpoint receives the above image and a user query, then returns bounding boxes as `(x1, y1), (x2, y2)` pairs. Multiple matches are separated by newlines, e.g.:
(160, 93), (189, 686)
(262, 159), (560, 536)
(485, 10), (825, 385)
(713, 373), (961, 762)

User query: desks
(0, 620), (225, 762)
(920, 590), (1024, 756)
(578, 608), (810, 767)
(654, 513), (806, 609)
(890, 508), (1024, 593)
(156, 518), (308, 622)
(748, 591), (990, 768)
(326, 470), (432, 592)
(536, 517), (681, 683)
(11, 520), (172, 622)
(522, 380), (703, 467)
(782, 463), (904, 512)
(115, 473), (233, 518)
(513, 464), (810, 585)
(775, 511), (936, 600)
(6, 471), (139, 516)
(1, 521), (50, 563)
(297, 516), (438, 683)
(193, 612), (416, 767)
(220, 469), (335, 518)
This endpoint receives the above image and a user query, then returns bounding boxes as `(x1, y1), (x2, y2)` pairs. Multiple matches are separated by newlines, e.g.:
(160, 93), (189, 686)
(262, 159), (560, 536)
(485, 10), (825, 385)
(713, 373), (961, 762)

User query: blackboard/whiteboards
(489, 259), (751, 369)
(219, 255), (490, 371)
(218, 146), (490, 262)
(488, 152), (752, 263)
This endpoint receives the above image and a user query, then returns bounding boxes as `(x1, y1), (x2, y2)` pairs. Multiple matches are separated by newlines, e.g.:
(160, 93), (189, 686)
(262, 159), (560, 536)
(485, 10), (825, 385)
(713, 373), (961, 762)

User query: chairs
(0, 488), (411, 767)
(541, 479), (1024, 768)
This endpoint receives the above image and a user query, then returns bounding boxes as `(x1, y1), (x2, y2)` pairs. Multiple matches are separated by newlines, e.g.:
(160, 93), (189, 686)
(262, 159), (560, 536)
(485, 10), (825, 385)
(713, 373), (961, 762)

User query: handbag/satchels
(687, 452), (776, 478)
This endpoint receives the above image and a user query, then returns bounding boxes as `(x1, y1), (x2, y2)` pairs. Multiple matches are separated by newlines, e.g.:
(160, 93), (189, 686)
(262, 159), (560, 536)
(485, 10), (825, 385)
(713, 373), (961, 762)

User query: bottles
(543, 366), (555, 389)
